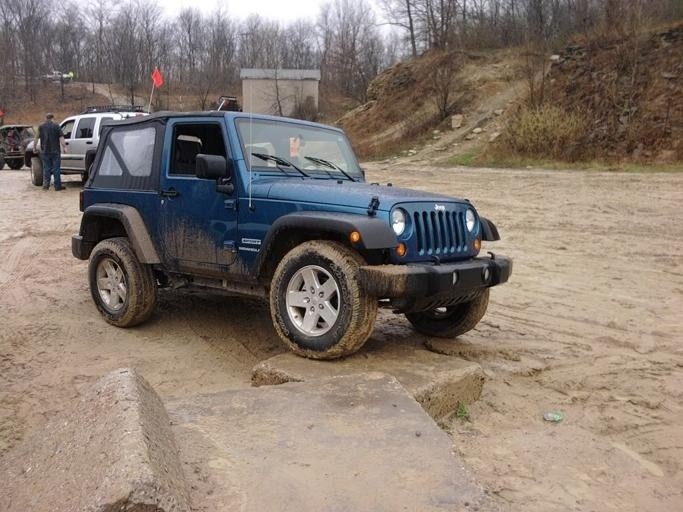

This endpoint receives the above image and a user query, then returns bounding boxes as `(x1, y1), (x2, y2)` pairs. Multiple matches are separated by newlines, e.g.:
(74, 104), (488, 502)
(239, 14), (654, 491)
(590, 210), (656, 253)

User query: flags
(151, 68), (163, 89)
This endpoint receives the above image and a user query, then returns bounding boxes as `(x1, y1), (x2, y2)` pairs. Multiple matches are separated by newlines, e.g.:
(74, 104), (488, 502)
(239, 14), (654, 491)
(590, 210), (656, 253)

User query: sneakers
(43, 186), (66, 191)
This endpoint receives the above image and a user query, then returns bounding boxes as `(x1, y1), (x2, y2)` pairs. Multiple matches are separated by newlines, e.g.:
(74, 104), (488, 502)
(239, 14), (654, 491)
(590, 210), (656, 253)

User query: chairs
(174, 139), (201, 176)
(243, 145), (268, 167)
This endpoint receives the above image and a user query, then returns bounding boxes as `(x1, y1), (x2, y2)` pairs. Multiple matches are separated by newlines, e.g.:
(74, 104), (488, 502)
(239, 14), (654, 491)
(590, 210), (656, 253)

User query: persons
(31, 112), (68, 192)
(5, 129), (18, 153)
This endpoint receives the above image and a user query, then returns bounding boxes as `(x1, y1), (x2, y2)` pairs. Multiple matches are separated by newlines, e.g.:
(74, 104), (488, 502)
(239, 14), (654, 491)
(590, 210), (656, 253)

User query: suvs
(73, 112), (517, 361)
(1, 122), (44, 169)
(29, 111), (149, 183)
